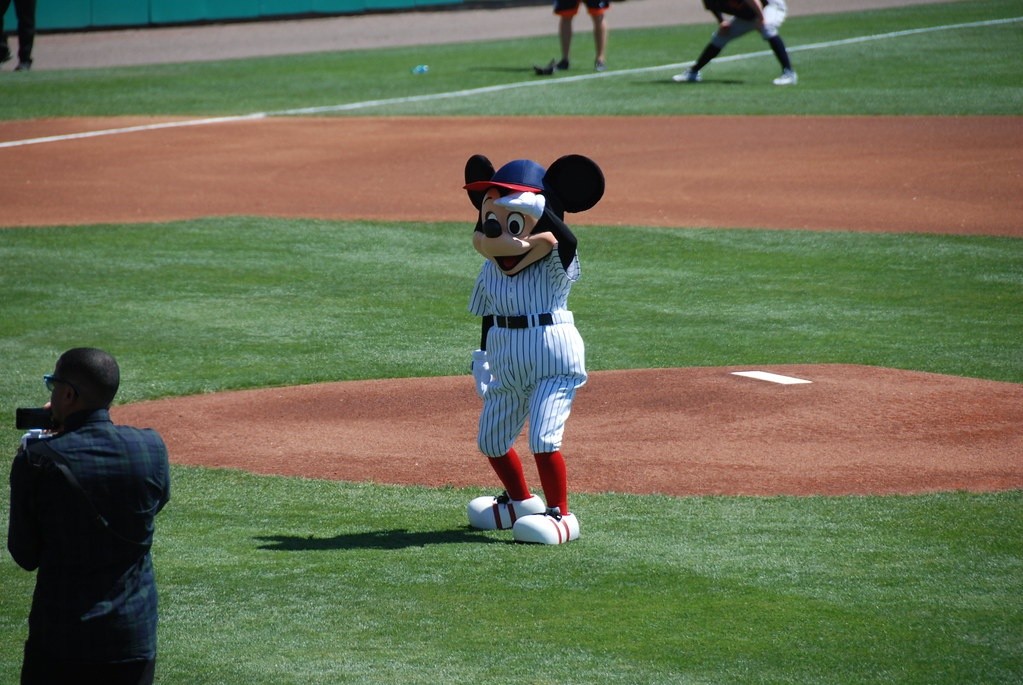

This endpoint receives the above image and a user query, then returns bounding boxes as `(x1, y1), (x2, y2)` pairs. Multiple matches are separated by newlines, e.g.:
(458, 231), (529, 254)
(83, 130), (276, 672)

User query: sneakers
(770, 69), (799, 86)
(595, 57), (608, 74)
(673, 70), (703, 84)
(551, 60), (573, 71)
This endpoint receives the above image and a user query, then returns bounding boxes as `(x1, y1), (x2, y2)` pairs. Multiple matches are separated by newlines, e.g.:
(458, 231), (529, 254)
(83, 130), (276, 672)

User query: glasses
(43, 375), (80, 394)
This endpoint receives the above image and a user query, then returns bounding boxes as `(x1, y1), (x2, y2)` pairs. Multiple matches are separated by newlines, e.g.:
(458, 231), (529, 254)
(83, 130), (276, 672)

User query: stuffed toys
(461, 154), (607, 546)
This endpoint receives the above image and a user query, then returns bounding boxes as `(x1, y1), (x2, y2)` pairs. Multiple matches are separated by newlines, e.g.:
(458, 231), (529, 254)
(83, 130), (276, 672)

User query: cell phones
(16, 408), (58, 430)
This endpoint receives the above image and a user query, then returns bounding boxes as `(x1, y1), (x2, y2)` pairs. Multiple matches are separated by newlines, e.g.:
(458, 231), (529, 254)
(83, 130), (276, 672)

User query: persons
(551, 0), (611, 73)
(5, 346), (170, 685)
(671, 0), (800, 87)
(0, 0), (38, 72)
